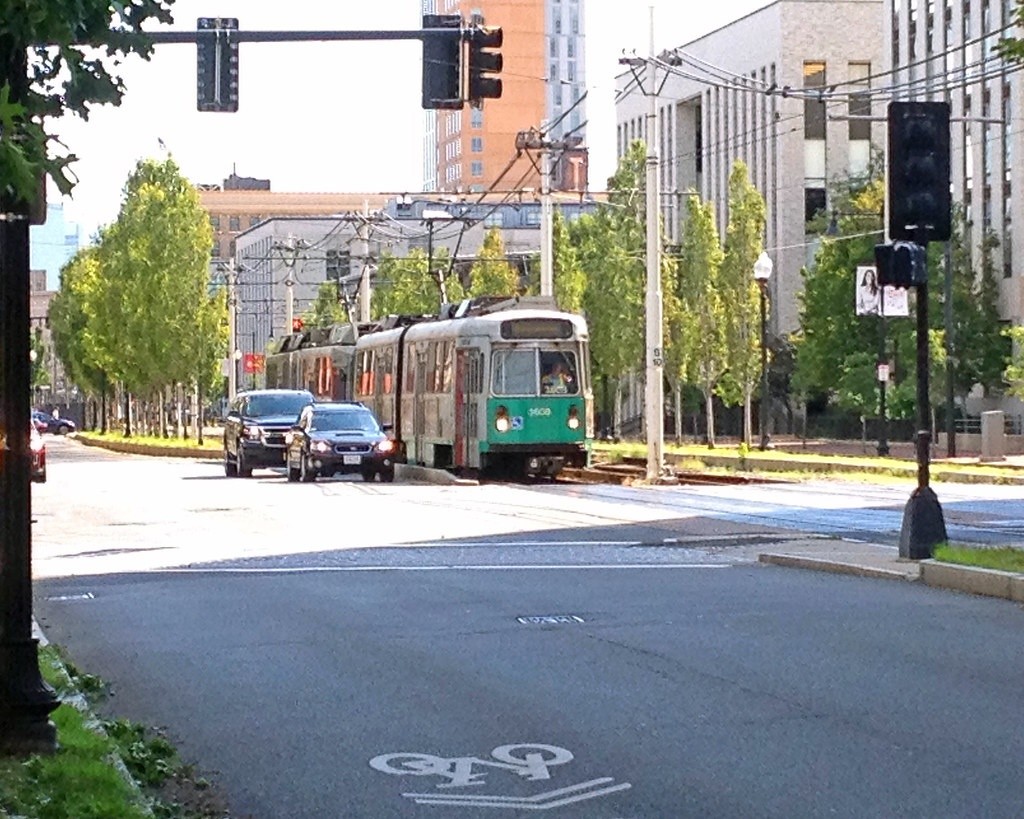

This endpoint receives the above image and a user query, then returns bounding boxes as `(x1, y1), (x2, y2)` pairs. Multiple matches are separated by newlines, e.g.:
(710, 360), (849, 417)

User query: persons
(857, 269), (883, 317)
(541, 362), (573, 395)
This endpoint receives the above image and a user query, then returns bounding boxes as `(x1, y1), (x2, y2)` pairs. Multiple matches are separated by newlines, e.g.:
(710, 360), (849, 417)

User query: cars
(33, 412), (75, 434)
(34, 418), (48, 436)
(31, 425), (46, 484)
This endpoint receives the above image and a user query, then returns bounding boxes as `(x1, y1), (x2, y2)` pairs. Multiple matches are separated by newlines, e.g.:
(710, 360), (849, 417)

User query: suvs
(223, 389), (317, 477)
(285, 401), (397, 483)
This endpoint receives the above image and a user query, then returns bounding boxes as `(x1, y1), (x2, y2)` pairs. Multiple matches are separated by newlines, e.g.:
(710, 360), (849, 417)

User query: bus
(265, 295), (595, 484)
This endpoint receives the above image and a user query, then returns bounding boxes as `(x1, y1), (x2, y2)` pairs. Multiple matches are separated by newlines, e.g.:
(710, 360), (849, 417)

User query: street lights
(30, 350), (38, 406)
(232, 347), (242, 396)
(751, 250), (774, 449)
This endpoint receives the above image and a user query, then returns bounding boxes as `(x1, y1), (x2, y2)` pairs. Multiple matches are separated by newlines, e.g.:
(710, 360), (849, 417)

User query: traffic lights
(467, 13), (502, 109)
(292, 318), (301, 331)
(885, 98), (952, 242)
